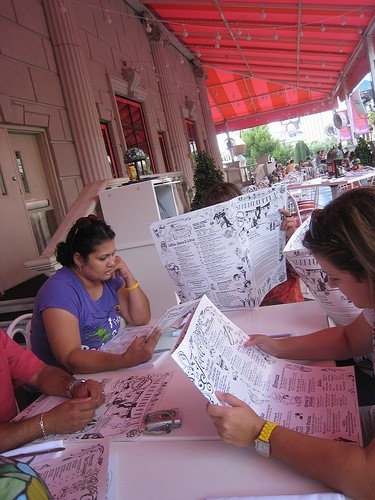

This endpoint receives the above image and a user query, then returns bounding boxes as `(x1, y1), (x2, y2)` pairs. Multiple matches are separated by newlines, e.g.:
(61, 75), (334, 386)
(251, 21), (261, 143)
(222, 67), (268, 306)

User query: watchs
(66, 379), (86, 399)
(254, 422), (277, 459)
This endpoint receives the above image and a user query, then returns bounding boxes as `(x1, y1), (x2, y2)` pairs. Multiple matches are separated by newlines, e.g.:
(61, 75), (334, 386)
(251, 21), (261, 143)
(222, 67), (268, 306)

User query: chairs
(6, 162), (375, 417)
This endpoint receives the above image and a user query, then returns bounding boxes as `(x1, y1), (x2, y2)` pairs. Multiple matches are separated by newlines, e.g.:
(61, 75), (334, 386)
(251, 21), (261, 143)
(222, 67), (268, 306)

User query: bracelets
(124, 281), (138, 291)
(39, 412), (47, 439)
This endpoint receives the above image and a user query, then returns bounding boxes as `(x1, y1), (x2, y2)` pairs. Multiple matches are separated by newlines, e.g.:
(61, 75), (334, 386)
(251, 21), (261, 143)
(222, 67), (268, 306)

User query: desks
(288, 170), (375, 211)
(11, 301), (361, 500)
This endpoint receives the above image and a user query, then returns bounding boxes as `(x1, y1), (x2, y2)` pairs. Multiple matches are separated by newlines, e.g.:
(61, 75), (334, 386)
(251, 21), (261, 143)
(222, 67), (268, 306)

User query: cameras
(144, 408), (182, 432)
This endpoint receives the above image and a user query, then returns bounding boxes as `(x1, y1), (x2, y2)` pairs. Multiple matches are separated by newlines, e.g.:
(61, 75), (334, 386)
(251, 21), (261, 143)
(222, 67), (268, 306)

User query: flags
(337, 111), (350, 140)
(350, 92), (370, 135)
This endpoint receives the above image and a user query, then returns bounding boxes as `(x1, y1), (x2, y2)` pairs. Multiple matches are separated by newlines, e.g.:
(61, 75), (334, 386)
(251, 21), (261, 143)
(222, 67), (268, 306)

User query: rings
(102, 391), (105, 395)
(286, 214), (293, 217)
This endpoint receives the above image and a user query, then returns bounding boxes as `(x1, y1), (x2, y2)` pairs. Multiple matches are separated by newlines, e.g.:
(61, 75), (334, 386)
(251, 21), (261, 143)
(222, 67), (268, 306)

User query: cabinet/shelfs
(96, 177), (184, 322)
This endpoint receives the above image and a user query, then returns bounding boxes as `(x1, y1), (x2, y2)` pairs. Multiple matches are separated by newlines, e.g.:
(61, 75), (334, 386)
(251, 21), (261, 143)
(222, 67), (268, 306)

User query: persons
(206, 186), (375, 500)
(0, 328), (106, 454)
(273, 150), (355, 180)
(202, 182), (304, 306)
(30, 214), (164, 374)
